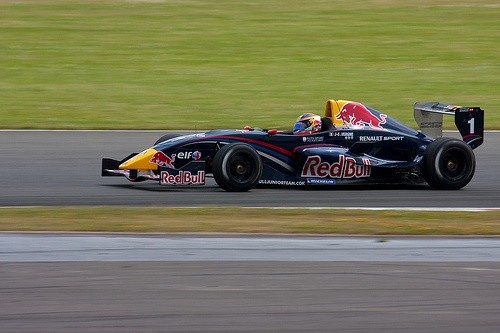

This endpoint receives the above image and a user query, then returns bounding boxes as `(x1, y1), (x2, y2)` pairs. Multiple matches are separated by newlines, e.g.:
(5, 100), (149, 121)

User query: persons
(294, 112), (322, 135)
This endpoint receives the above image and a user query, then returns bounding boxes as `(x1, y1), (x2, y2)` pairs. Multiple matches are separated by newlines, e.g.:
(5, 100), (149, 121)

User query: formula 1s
(99, 97), (486, 191)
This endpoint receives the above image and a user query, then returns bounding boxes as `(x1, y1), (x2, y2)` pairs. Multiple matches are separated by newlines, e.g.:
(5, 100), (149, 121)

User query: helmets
(293, 113), (321, 135)
